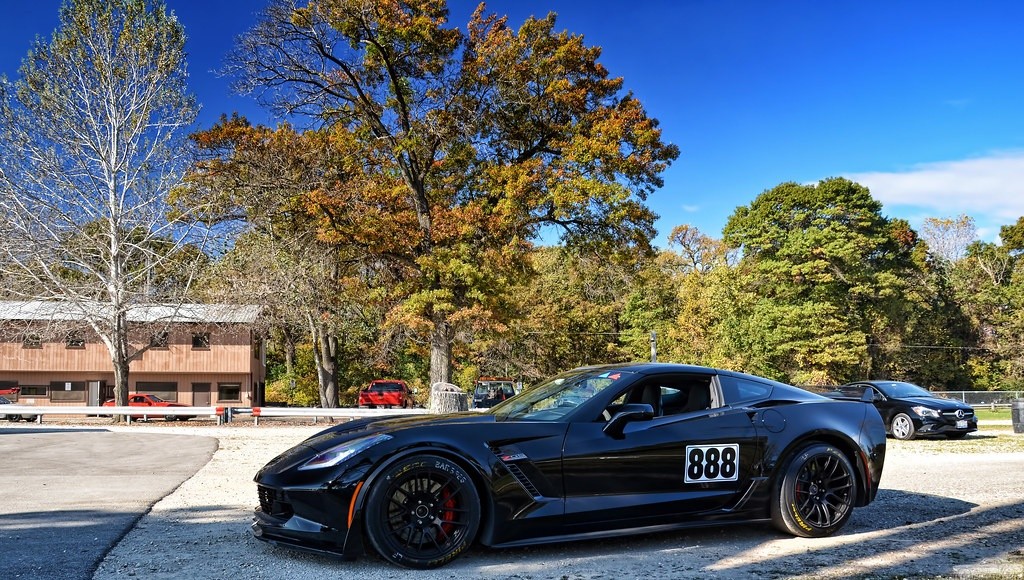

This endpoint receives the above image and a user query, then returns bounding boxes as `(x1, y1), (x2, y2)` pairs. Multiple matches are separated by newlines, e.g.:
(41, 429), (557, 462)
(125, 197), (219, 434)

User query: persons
(488, 387), (504, 408)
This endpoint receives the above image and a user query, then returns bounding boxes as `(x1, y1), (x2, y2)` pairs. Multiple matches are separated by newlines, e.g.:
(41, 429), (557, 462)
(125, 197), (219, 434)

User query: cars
(836, 380), (979, 441)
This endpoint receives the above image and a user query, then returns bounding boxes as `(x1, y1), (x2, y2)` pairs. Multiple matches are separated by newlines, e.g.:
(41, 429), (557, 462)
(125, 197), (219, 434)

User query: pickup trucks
(358, 380), (418, 409)
(471, 377), (516, 409)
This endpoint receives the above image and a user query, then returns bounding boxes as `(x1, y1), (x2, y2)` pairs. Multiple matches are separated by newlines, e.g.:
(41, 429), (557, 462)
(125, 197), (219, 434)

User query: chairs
(641, 382), (663, 417)
(687, 382), (709, 411)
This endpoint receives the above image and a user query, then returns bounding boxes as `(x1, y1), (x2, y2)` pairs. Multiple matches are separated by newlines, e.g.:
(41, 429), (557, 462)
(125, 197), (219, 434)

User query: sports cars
(0, 395), (37, 422)
(249, 358), (888, 569)
(102, 394), (197, 420)
